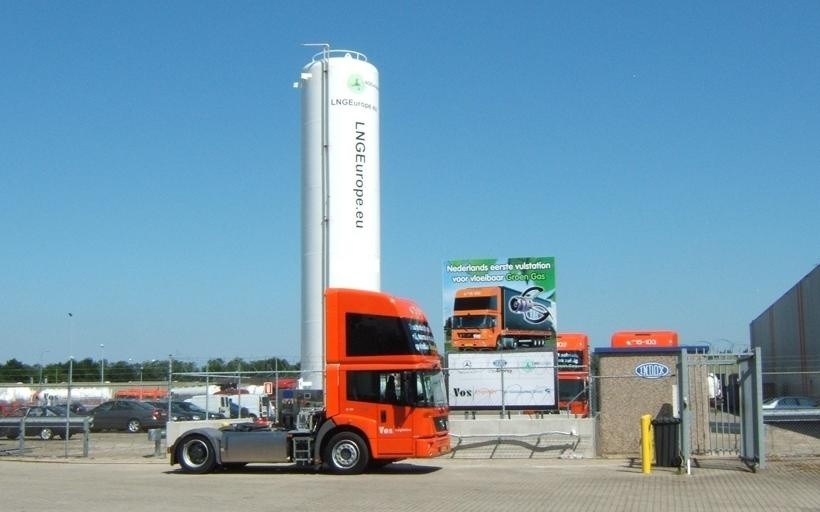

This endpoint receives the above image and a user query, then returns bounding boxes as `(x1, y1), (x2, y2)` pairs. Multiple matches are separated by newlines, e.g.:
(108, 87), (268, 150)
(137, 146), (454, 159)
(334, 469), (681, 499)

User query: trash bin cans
(652, 418), (682, 467)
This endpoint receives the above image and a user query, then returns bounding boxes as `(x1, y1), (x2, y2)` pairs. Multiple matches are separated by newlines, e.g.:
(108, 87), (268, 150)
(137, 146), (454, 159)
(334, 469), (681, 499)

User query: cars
(762, 393), (820, 423)
(0, 399), (94, 441)
(87, 397), (224, 433)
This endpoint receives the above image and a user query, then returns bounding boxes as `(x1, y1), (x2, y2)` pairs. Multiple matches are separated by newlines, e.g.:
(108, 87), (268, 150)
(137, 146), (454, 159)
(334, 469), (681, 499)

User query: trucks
(521, 332), (591, 414)
(166, 289), (451, 475)
(450, 286), (552, 350)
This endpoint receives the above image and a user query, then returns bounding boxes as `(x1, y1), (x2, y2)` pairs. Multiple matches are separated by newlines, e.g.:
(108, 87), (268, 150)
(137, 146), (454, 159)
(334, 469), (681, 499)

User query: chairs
(386, 376), (396, 401)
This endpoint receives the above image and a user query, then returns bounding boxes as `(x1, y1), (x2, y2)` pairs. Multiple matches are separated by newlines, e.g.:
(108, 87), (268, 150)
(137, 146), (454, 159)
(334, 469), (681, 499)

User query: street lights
(69, 355), (74, 383)
(140, 367), (144, 381)
(100, 344), (104, 382)
